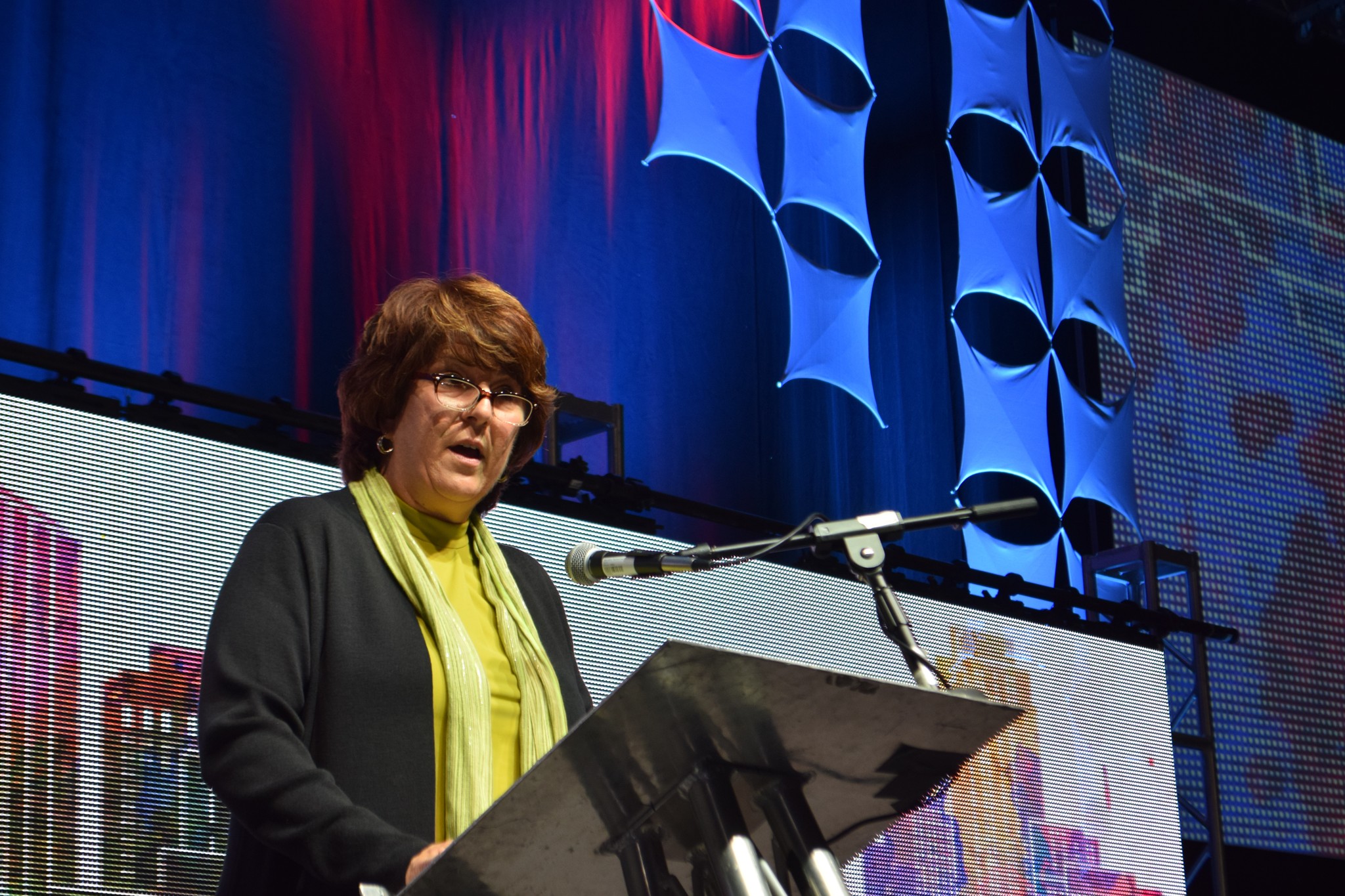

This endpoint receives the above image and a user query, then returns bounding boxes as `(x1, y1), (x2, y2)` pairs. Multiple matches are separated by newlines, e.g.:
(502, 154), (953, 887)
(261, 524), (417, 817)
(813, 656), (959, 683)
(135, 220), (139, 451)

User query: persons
(197, 268), (593, 896)
(1254, 405), (1345, 856)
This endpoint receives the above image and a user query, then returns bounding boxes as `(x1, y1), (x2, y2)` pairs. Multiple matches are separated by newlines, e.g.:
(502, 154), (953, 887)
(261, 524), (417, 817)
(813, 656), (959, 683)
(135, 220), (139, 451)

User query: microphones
(564, 543), (709, 585)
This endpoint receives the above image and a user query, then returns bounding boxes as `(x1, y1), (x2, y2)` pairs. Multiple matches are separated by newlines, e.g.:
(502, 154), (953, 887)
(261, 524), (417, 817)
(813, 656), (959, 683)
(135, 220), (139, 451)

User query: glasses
(416, 372), (539, 427)
